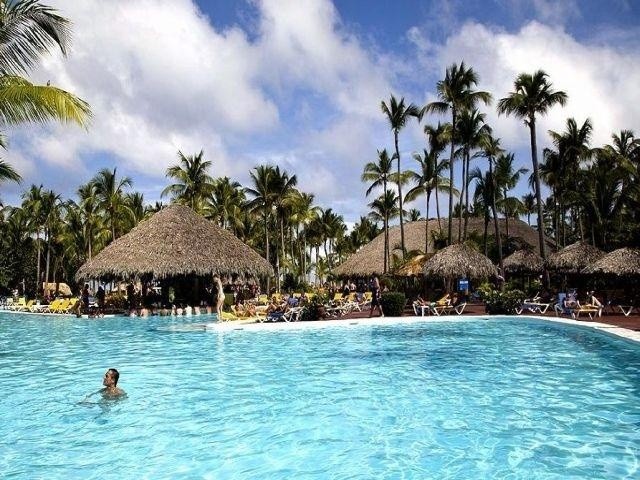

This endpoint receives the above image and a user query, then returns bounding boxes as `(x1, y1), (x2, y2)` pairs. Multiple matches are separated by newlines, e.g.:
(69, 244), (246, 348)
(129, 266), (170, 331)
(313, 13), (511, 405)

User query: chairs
(0, 296), (80, 316)
(515, 291), (640, 320)
(217, 288), (471, 325)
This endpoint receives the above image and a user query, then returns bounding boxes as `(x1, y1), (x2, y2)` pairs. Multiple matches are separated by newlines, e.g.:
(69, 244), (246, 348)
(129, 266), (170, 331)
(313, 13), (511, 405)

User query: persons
(80, 283), (93, 314)
(94, 286), (105, 316)
(205, 275), (606, 323)
(100, 366), (126, 400)
